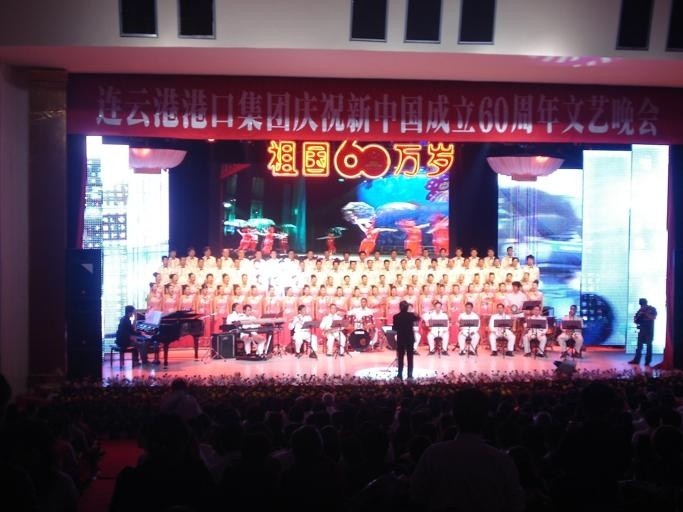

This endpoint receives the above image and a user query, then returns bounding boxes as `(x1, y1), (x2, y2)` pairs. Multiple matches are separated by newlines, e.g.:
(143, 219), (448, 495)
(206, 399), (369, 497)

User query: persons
(626, 297), (658, 366)
(115, 305), (151, 365)
(394, 303), (420, 355)
(223, 302), (259, 329)
(454, 302), (480, 355)
(555, 305), (584, 358)
(144, 246), (544, 333)
(318, 303), (346, 357)
(235, 217), (449, 256)
(286, 305), (317, 357)
(487, 303), (516, 357)
(423, 302), (450, 356)
(237, 303), (266, 358)
(391, 300), (420, 379)
(346, 298), (377, 347)
(521, 305), (547, 357)
(0, 369), (682, 512)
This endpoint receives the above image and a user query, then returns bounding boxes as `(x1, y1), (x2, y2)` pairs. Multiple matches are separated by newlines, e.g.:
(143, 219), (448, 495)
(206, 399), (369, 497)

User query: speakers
(68, 248), (102, 381)
(212, 333), (235, 358)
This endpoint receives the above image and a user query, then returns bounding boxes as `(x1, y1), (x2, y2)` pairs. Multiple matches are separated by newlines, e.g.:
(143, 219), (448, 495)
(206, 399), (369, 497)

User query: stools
(109, 344), (139, 371)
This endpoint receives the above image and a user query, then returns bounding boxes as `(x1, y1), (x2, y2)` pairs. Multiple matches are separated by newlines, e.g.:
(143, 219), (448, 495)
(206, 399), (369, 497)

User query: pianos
(132, 309), (203, 369)
(219, 318), (287, 332)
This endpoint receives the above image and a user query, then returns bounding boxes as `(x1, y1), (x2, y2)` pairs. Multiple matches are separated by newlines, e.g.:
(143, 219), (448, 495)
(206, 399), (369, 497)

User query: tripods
(272, 329), (352, 358)
(385, 349), (399, 372)
(428, 329), (582, 360)
(201, 318), (227, 362)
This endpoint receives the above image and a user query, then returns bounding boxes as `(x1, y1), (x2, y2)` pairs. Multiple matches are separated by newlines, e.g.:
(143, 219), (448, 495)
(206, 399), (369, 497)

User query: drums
(348, 329), (370, 352)
(343, 314), (356, 321)
(363, 316), (373, 321)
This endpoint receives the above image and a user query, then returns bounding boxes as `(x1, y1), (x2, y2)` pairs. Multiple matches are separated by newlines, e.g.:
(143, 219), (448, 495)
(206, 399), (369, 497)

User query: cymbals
(372, 303), (391, 308)
